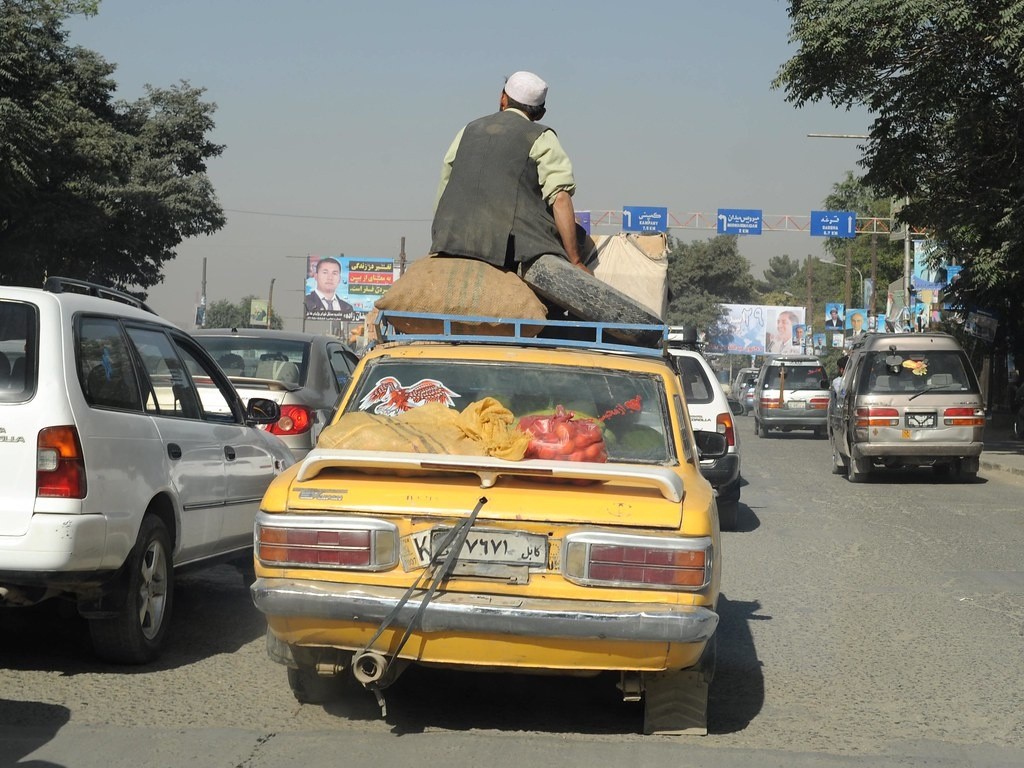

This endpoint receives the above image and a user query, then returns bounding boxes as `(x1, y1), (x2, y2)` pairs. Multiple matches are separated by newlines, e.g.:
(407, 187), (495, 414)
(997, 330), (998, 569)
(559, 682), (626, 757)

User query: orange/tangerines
(525, 423), (606, 462)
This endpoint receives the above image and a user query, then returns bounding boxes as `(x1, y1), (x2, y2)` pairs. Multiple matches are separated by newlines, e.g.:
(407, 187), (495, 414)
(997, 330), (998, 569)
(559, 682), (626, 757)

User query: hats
(504, 71), (548, 107)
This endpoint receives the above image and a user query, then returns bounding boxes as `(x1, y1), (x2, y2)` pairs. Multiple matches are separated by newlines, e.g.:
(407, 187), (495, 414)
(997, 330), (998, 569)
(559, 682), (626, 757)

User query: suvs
(0, 277), (297, 664)
(138, 328), (360, 463)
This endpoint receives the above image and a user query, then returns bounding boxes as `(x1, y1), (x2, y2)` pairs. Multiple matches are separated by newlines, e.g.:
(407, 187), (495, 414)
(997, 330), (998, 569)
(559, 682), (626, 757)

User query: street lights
(820, 259), (863, 308)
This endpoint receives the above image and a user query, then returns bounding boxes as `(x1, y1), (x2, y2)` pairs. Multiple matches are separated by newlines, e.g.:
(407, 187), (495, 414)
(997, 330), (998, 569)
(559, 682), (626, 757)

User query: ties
(780, 344), (785, 352)
(854, 332), (857, 336)
(322, 297), (335, 310)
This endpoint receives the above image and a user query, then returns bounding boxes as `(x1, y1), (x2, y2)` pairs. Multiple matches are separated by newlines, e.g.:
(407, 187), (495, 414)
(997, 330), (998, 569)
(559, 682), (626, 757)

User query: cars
(250, 309), (722, 736)
(588, 348), (744, 531)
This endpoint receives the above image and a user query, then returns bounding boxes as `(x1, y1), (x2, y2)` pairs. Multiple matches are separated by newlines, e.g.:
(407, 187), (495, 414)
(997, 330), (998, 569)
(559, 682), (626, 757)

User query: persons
(305, 258), (354, 310)
(427, 71), (593, 347)
(832, 357), (850, 392)
(770, 310), (867, 356)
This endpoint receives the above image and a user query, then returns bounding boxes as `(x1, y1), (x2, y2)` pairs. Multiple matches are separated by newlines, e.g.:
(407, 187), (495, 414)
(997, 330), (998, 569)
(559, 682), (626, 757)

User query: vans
(718, 356), (831, 438)
(828, 332), (985, 483)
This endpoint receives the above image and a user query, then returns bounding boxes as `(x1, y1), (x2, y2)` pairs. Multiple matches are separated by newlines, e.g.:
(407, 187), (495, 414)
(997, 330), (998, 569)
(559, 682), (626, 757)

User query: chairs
(805, 377), (817, 383)
(773, 378), (787, 388)
(931, 374), (953, 386)
(874, 376), (902, 392)
(156, 358), (196, 376)
(88, 362), (149, 410)
(258, 360), (298, 386)
(221, 368), (246, 377)
